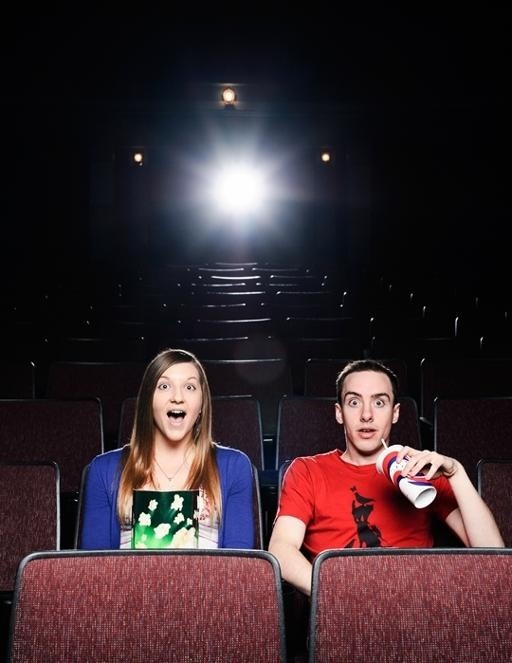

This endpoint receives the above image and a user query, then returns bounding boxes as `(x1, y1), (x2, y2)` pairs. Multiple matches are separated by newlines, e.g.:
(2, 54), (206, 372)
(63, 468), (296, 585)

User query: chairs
(14, 549), (286, 661)
(307, 548), (511, 659)
(2, 253), (512, 549)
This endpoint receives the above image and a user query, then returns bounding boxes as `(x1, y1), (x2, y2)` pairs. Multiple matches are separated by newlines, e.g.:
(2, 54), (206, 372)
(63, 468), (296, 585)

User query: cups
(376, 438), (437, 509)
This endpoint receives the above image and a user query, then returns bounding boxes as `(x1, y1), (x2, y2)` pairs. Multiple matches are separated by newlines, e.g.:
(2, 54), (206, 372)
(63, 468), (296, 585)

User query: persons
(77, 341), (255, 551)
(267, 357), (511, 606)
